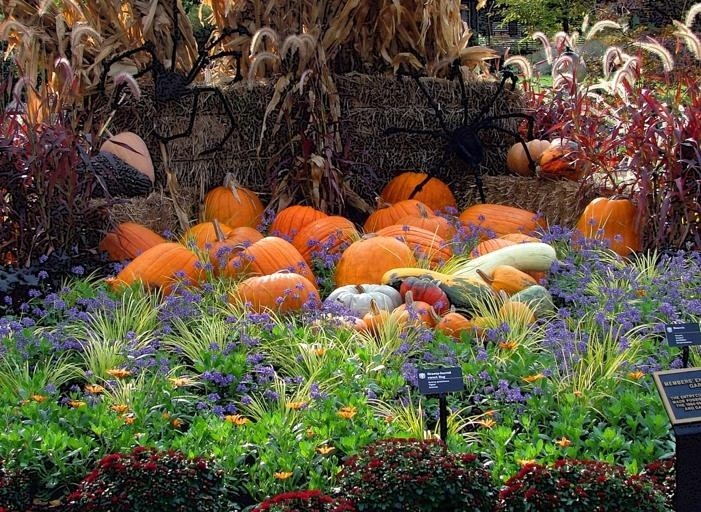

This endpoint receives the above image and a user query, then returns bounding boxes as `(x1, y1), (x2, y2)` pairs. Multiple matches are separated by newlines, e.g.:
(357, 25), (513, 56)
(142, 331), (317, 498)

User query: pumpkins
(97, 139), (647, 336)
